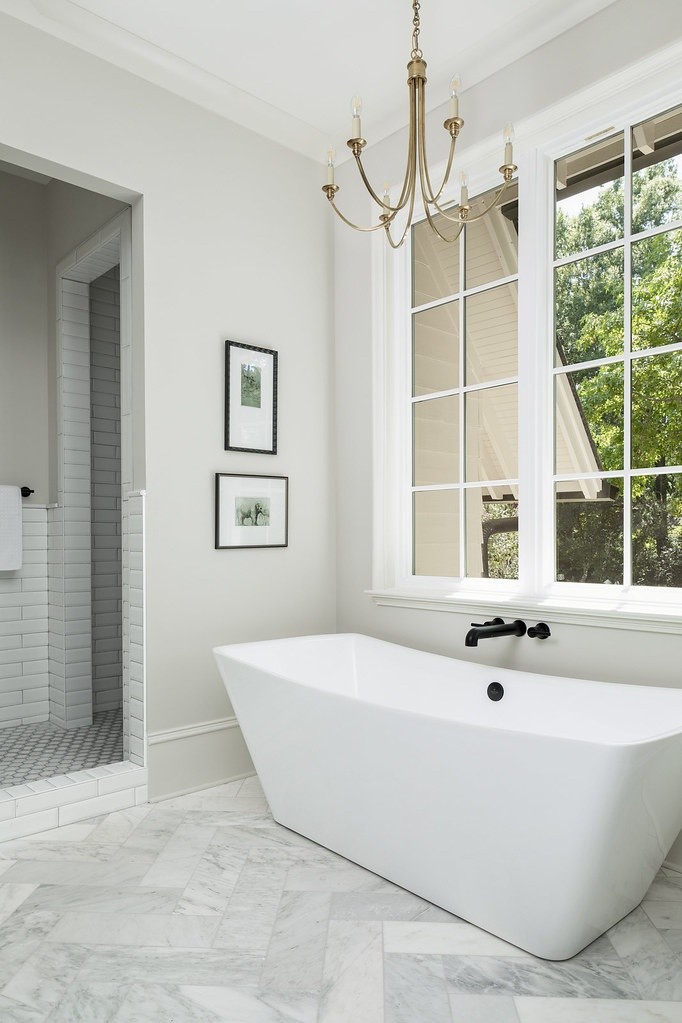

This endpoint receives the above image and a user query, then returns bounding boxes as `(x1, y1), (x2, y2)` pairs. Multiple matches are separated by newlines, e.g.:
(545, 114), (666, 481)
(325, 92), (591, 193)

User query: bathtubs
(210, 630), (682, 963)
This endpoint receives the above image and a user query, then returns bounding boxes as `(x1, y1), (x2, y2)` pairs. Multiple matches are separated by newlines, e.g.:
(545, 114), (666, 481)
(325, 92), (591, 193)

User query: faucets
(464, 618), (528, 647)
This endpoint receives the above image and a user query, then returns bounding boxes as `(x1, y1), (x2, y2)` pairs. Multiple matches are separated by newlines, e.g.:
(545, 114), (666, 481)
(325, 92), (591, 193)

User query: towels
(0, 484), (23, 572)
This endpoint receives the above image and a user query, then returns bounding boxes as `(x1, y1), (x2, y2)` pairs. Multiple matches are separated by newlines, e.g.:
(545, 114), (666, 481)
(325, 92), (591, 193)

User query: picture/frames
(225, 340), (279, 456)
(216, 472), (289, 548)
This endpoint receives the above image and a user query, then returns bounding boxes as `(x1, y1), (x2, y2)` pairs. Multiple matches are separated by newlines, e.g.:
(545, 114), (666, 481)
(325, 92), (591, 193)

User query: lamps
(323, 0), (516, 249)
